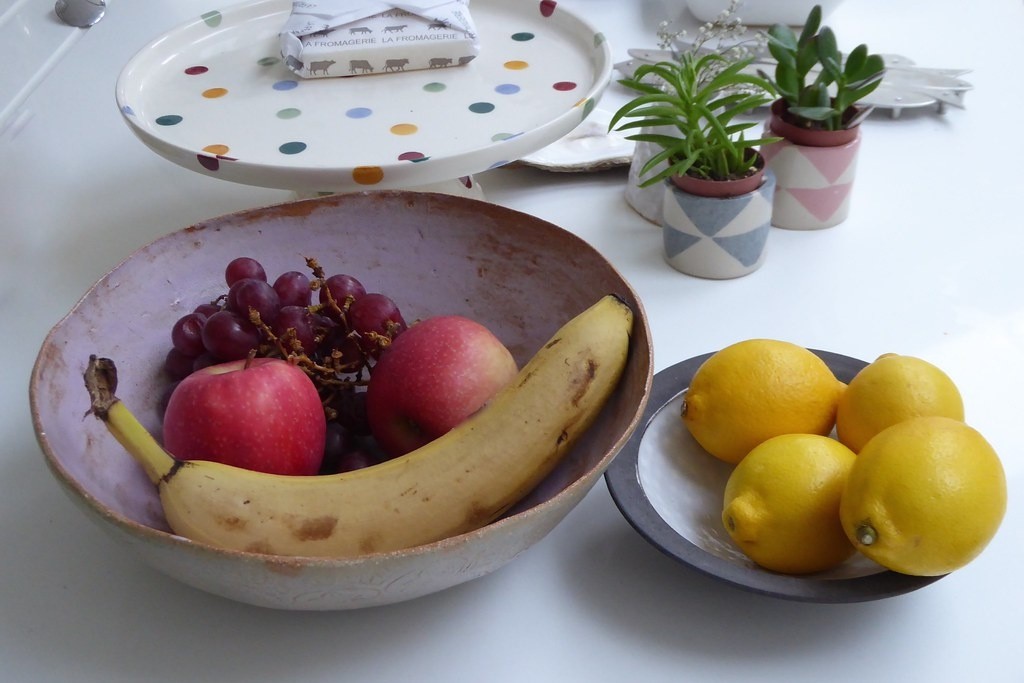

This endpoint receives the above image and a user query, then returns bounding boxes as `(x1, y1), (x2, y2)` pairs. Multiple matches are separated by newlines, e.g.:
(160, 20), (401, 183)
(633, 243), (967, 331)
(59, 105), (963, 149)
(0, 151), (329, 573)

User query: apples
(366, 315), (518, 456)
(162, 348), (326, 477)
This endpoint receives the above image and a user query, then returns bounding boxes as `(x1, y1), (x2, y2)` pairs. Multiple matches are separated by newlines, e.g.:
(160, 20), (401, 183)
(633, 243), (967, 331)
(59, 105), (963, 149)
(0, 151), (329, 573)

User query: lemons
(835, 352), (964, 453)
(721, 432), (863, 575)
(838, 415), (1007, 576)
(679, 339), (848, 461)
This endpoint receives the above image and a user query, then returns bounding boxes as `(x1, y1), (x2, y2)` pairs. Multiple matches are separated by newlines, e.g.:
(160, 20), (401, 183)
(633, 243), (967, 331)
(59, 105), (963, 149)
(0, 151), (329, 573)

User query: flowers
(626, 0), (769, 96)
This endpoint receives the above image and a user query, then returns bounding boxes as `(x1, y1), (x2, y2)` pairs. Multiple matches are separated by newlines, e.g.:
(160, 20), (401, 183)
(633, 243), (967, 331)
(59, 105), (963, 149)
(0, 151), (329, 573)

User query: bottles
(627, 28), (727, 224)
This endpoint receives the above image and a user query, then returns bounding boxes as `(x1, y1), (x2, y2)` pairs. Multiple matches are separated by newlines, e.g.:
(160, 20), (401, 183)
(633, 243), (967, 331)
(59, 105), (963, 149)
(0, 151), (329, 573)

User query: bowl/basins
(30, 187), (653, 616)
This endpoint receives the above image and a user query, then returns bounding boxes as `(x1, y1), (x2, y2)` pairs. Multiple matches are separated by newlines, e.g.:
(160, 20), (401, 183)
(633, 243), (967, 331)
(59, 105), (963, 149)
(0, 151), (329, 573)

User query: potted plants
(757, 4), (886, 231)
(607, 51), (784, 278)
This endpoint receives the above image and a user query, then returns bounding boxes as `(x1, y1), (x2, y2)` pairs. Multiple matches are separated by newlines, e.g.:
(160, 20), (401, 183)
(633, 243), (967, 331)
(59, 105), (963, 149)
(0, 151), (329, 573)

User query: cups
(684, 0), (842, 28)
(757, 126), (860, 231)
(660, 169), (779, 282)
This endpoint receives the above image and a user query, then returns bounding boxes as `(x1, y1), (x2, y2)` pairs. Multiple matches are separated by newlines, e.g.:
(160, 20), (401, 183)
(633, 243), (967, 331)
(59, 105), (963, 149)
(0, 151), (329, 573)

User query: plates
(603, 345), (952, 604)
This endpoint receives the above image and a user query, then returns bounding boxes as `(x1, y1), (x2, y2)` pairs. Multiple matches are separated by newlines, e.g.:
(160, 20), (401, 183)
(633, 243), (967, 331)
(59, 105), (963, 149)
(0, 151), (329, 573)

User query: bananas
(82, 293), (634, 558)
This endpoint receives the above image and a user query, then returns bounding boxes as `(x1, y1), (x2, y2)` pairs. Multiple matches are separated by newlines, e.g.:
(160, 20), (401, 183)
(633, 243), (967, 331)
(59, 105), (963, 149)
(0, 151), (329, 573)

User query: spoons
(55, 0), (106, 28)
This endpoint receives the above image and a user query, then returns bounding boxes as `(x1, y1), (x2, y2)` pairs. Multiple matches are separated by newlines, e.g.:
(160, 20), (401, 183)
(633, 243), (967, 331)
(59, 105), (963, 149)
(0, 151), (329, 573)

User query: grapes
(165, 257), (406, 474)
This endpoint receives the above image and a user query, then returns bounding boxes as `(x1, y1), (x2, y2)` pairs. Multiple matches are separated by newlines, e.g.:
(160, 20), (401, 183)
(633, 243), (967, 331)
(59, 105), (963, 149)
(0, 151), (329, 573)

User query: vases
(626, 102), (725, 227)
